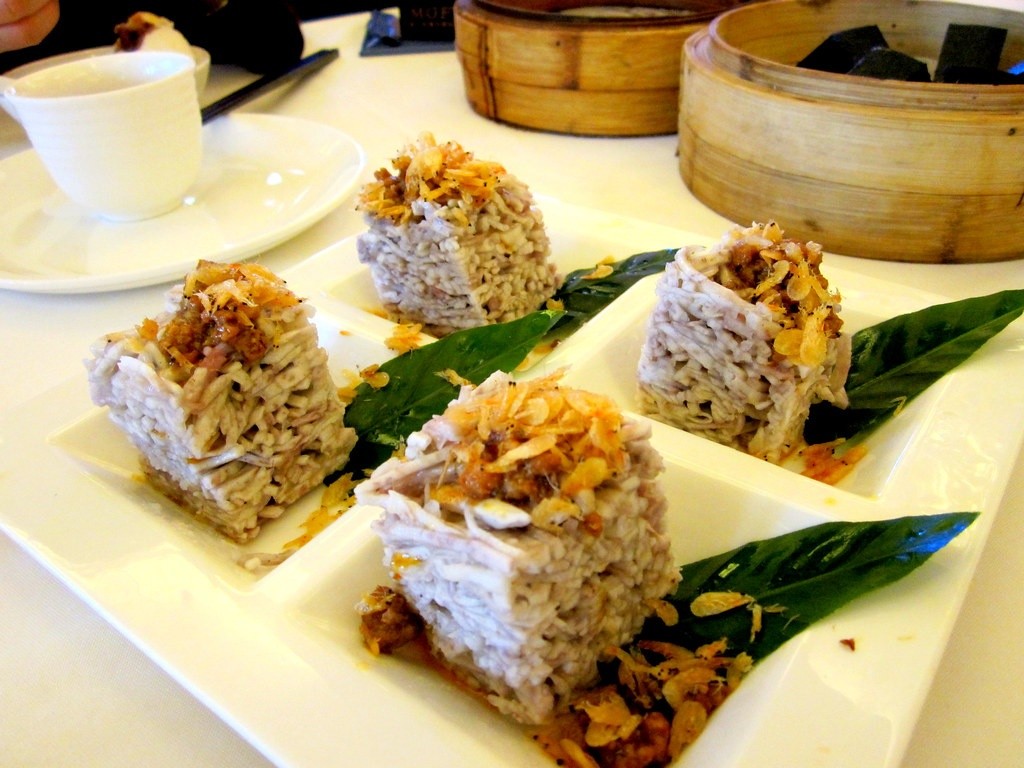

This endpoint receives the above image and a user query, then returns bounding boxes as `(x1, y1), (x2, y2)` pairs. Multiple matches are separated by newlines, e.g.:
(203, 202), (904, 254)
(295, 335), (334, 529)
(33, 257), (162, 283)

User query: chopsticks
(200, 47), (338, 126)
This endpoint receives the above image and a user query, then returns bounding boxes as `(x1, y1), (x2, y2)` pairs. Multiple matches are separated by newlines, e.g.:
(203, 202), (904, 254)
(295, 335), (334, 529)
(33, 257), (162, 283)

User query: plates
(1, 200), (1024, 768)
(1, 117), (365, 292)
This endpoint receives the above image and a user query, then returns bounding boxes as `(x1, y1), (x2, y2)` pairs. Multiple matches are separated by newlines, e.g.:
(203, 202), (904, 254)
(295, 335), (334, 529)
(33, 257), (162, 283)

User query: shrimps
(102, 132), (863, 768)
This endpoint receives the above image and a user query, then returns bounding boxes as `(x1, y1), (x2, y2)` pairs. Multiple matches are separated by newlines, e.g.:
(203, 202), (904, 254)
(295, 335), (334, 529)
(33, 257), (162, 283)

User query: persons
(0, 1), (304, 75)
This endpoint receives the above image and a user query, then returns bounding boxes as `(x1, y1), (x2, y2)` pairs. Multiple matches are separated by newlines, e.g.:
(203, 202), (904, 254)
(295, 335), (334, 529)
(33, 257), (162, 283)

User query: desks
(0, 51), (1024, 768)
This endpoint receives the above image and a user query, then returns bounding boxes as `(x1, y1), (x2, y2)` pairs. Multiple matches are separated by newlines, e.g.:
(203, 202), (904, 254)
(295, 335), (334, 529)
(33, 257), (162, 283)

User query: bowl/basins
(0, 45), (211, 127)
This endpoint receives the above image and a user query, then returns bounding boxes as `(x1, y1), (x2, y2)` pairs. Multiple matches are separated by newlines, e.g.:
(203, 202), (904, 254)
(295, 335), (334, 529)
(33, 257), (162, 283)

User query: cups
(6, 50), (204, 225)
